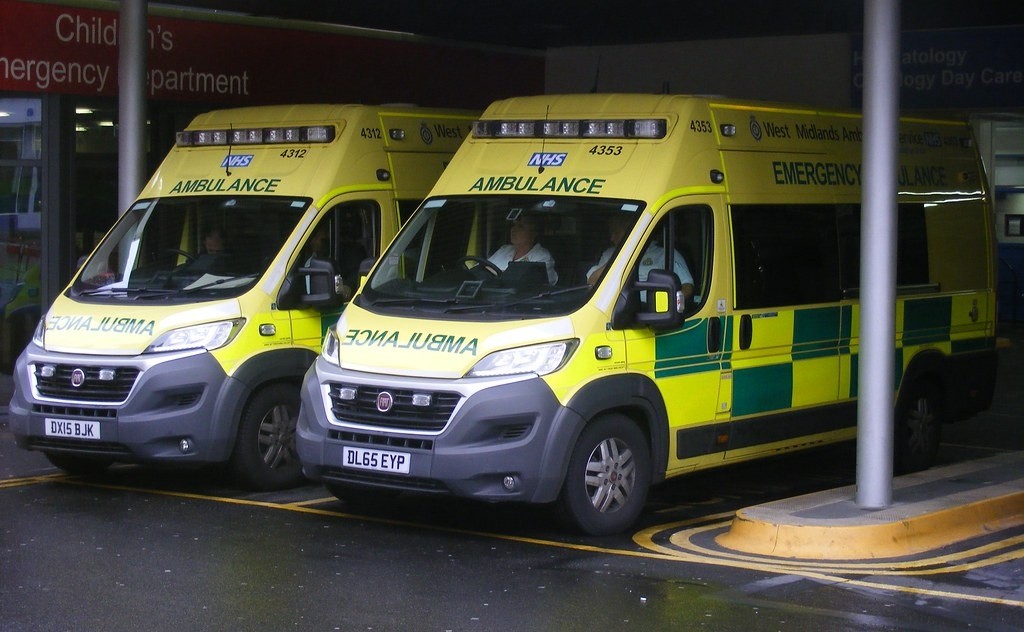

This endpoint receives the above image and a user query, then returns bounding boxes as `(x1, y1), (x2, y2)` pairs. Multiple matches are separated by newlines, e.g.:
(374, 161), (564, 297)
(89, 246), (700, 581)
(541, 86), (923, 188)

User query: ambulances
(0, 96), (197, 369)
(292, 93), (1003, 537)
(7, 101), (503, 492)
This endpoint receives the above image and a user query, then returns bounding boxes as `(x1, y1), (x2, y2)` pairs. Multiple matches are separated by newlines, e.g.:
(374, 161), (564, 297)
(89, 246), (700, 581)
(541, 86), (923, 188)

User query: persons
(303, 227), (348, 278)
(186, 227), (244, 272)
(472, 209), (558, 286)
(586, 210), (696, 309)
